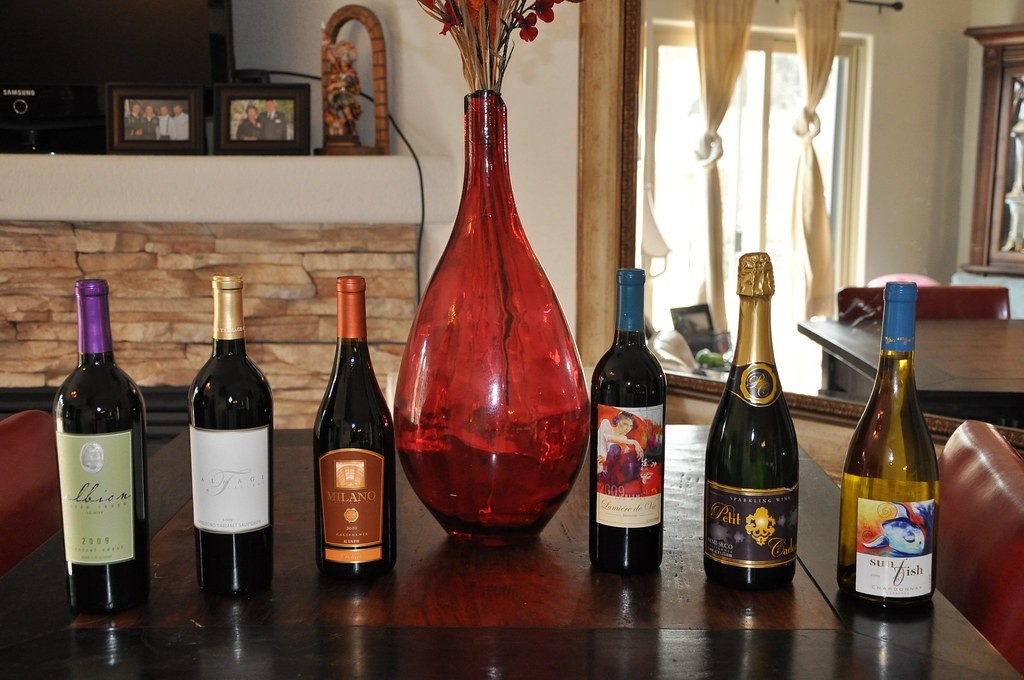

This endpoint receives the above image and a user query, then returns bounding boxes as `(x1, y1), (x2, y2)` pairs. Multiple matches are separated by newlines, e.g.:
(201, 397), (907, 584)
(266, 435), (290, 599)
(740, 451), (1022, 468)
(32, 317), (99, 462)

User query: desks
(0, 428), (1022, 680)
(798, 319), (1024, 430)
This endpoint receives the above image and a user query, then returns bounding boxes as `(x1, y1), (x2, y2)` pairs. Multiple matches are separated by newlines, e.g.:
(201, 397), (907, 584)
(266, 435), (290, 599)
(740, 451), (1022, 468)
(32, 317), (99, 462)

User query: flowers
(414, 0), (581, 407)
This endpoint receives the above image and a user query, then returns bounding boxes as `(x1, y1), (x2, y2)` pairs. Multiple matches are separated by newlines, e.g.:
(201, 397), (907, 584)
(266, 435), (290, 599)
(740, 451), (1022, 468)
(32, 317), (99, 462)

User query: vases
(392, 90), (588, 549)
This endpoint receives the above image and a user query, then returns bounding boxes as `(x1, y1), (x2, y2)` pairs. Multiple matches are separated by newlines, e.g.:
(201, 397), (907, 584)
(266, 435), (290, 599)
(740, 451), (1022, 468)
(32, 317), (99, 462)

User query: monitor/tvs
(0, 0), (237, 153)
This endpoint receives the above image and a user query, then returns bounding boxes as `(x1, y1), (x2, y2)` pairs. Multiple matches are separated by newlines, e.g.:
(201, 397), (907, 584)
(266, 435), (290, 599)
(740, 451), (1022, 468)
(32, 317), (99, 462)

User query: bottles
(837, 281), (941, 605)
(314, 275), (396, 580)
(188, 274), (275, 595)
(587, 269), (668, 575)
(702, 252), (800, 591)
(51, 278), (149, 614)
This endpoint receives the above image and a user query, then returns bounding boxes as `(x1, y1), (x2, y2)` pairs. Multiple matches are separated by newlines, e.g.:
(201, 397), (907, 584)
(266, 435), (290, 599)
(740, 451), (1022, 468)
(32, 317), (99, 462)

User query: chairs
(936, 420), (1024, 678)
(836, 286), (1010, 323)
(0, 410), (58, 523)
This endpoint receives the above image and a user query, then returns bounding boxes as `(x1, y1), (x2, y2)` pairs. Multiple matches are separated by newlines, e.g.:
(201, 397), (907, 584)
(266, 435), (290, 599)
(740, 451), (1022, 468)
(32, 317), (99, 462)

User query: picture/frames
(212, 83), (311, 156)
(104, 82), (208, 156)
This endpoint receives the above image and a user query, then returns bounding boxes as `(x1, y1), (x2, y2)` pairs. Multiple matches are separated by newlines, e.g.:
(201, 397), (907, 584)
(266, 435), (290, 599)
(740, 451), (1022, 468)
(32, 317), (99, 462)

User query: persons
(236, 101), (288, 140)
(124, 101), (189, 139)
(328, 52), (362, 147)
